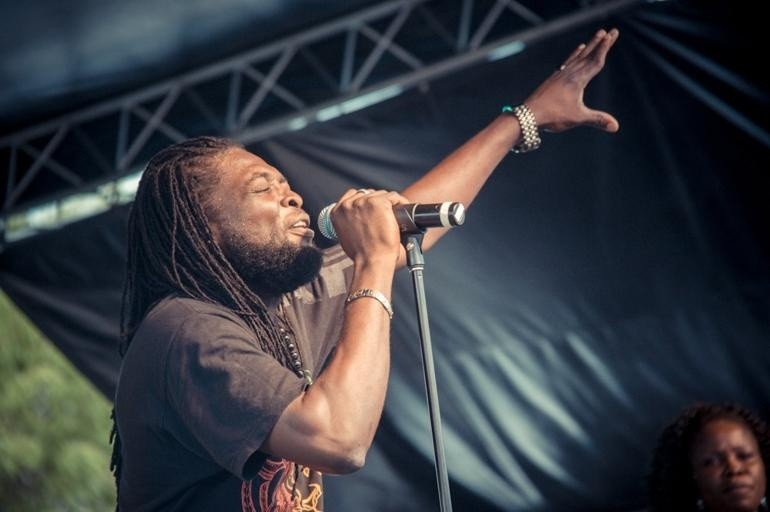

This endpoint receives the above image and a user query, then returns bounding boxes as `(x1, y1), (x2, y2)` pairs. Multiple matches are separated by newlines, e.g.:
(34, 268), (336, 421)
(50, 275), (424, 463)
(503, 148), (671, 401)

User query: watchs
(502, 104), (542, 153)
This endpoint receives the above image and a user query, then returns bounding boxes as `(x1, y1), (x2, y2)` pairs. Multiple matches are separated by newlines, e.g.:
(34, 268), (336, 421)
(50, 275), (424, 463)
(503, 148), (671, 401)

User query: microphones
(317, 201), (466, 240)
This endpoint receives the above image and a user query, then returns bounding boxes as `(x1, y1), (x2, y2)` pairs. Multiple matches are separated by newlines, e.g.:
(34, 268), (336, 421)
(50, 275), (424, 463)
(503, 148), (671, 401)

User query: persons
(110, 27), (622, 512)
(655, 404), (770, 512)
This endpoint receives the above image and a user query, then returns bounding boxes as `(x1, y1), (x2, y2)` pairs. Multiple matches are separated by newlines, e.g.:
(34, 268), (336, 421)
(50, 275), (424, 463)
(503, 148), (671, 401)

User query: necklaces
(276, 325), (303, 377)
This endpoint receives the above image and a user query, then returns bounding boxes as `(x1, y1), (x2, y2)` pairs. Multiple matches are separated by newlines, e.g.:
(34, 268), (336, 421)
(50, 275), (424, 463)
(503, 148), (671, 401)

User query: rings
(560, 65), (566, 71)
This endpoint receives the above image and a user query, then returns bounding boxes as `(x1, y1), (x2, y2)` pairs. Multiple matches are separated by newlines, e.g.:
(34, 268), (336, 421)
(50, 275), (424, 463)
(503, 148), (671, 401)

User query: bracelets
(344, 288), (393, 318)
